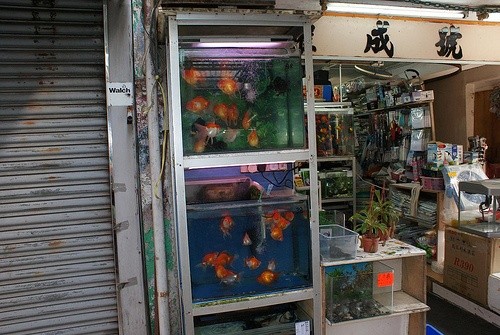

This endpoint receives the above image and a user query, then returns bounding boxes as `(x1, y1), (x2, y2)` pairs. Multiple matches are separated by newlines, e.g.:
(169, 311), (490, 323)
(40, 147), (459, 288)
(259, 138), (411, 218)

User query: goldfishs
(317, 115), (354, 145)
(181, 67), (291, 153)
(194, 209), (294, 285)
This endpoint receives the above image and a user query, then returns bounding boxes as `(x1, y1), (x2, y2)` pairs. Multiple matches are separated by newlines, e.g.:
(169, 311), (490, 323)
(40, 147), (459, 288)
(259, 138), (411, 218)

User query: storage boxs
(427, 141), (464, 165)
(442, 226), (500, 307)
(154, 6), (430, 335)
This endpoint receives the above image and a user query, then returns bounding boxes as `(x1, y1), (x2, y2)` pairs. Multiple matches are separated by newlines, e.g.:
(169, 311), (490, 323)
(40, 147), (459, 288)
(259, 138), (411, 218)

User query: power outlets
(240, 162), (288, 173)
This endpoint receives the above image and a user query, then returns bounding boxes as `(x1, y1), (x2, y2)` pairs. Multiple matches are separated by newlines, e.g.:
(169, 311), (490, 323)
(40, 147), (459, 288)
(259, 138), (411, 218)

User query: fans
(354, 61), (393, 81)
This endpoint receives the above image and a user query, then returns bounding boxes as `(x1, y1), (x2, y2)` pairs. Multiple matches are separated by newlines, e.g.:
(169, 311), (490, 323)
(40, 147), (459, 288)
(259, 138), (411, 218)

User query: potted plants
(368, 188), (402, 241)
(348, 202), (390, 254)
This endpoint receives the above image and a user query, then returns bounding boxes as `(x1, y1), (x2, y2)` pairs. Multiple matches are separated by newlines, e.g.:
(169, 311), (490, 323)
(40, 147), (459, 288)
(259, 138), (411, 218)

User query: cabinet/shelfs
(388, 182), (459, 275)
